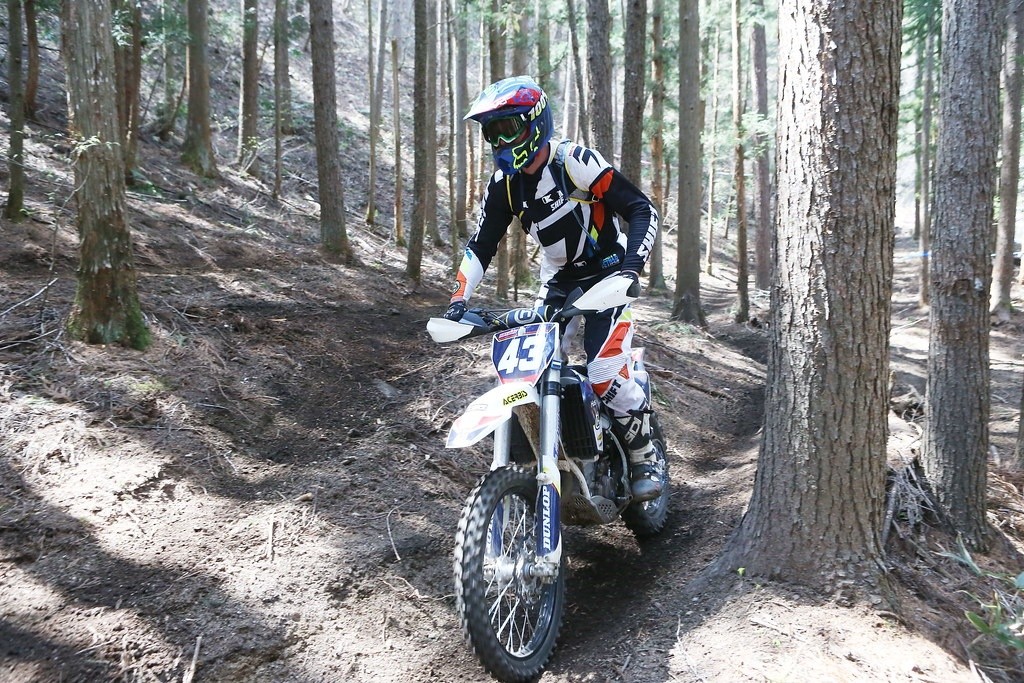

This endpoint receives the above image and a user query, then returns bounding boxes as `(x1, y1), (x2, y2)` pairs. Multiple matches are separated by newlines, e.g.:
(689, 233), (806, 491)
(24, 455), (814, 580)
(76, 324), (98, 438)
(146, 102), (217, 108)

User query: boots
(599, 399), (670, 535)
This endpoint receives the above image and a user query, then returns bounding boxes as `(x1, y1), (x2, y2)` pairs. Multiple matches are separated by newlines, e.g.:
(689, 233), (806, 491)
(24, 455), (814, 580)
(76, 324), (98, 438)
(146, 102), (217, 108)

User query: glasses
(482, 89), (549, 148)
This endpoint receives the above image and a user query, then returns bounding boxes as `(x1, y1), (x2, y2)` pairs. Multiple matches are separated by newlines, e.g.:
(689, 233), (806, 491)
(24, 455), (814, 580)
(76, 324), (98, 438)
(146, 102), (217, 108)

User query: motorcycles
(424, 269), (675, 683)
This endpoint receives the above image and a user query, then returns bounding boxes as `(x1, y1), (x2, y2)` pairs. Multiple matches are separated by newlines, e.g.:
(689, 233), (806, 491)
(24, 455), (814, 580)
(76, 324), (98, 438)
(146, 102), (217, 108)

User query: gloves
(442, 301), (468, 322)
(618, 270), (639, 285)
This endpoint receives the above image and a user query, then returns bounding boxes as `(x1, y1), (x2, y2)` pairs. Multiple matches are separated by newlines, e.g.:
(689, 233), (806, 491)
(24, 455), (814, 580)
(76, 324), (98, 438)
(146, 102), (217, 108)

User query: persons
(445, 75), (667, 502)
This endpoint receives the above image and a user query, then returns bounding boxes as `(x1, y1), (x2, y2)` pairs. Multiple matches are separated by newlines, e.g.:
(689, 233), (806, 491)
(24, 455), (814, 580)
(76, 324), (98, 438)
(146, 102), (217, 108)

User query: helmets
(462, 75), (554, 175)
